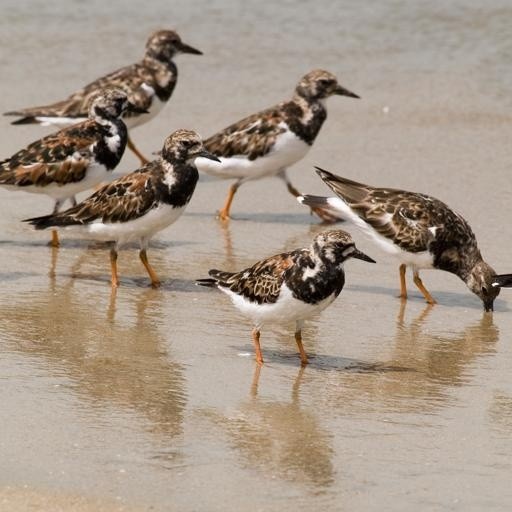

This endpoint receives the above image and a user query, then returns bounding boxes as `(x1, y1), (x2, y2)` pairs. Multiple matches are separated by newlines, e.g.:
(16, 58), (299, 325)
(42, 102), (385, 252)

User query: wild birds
(0, 89), (150, 247)
(172, 68), (360, 221)
(195, 230), (376, 366)
(19, 129), (222, 289)
(313, 166), (512, 312)
(2, 29), (204, 167)
